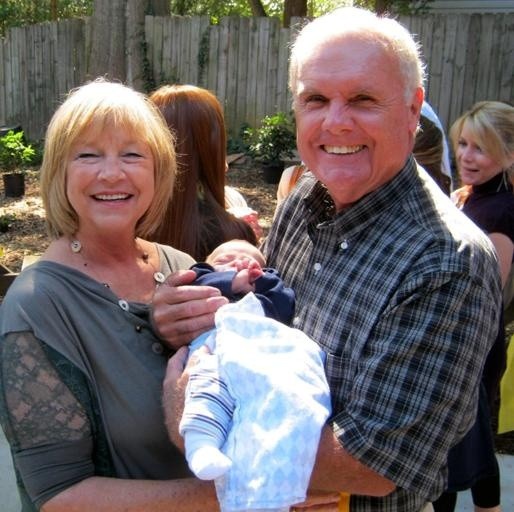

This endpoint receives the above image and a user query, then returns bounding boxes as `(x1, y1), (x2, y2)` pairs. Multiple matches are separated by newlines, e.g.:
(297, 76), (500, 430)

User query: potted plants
(0, 129), (37, 196)
(241, 111), (297, 184)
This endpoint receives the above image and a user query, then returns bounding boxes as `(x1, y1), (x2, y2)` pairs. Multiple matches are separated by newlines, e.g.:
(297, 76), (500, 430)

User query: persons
(134, 84), (261, 265)
(273, 98), (513, 511)
(175, 237), (334, 486)
(150, 5), (504, 511)
(0, 74), (343, 510)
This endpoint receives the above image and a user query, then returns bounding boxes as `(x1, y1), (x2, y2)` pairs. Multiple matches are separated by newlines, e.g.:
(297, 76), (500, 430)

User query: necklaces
(66, 231), (164, 357)
